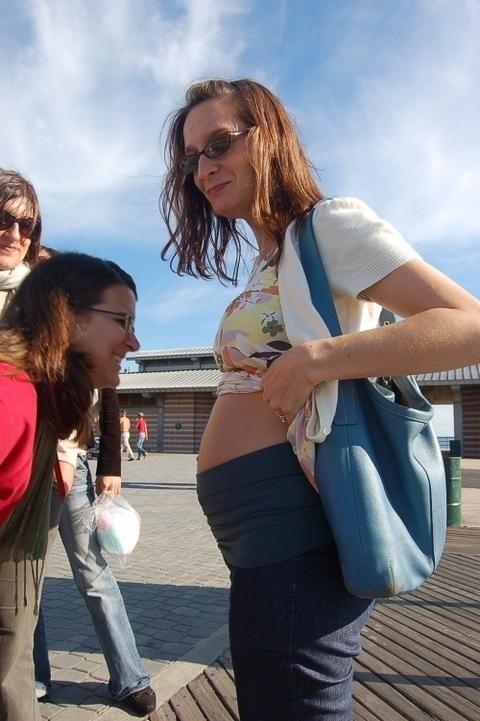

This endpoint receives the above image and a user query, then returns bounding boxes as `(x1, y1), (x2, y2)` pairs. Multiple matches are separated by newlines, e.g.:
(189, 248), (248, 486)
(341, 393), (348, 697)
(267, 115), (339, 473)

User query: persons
(154, 79), (480, 721)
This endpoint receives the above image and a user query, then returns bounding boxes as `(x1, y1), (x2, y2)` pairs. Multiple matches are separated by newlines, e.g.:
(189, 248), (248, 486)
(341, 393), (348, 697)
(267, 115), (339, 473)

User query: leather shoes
(127, 452), (148, 461)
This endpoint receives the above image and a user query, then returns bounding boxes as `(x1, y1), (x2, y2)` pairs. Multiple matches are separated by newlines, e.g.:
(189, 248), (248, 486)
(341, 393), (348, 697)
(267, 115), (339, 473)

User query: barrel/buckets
(444, 456), (461, 527)
(450, 440), (460, 457)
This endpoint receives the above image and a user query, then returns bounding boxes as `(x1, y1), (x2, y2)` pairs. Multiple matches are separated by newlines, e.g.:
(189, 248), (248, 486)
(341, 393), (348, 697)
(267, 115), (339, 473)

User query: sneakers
(124, 685), (157, 714)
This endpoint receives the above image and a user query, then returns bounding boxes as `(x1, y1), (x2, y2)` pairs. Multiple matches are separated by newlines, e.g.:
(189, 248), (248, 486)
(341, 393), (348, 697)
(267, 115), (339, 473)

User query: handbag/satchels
(296, 196), (447, 600)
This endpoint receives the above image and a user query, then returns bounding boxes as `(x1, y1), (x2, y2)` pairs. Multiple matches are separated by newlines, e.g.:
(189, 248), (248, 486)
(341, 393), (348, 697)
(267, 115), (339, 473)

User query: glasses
(84, 307), (136, 335)
(0, 209), (40, 238)
(178, 129), (250, 177)
